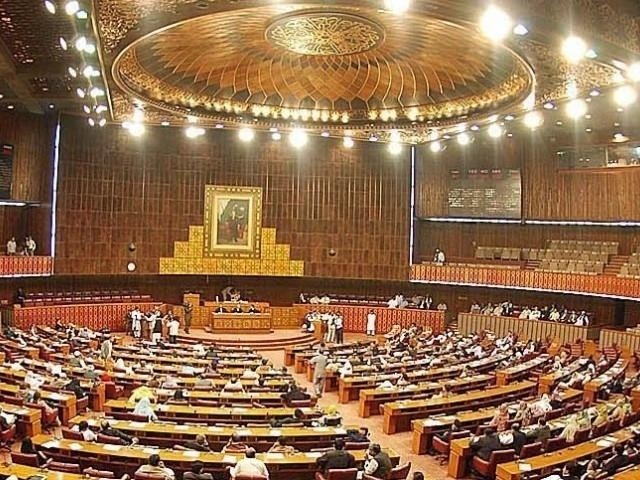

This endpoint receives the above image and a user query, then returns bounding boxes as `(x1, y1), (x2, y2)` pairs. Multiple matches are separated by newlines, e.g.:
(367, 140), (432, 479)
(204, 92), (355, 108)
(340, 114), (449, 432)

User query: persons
(434, 248), (444, 264)
(1, 237), (39, 258)
(2, 285), (640, 479)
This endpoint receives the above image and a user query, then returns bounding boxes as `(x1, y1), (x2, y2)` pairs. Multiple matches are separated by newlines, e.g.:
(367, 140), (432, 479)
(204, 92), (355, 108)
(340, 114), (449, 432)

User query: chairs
(476, 239), (640, 280)
(299, 291), (429, 308)
(23, 288), (157, 306)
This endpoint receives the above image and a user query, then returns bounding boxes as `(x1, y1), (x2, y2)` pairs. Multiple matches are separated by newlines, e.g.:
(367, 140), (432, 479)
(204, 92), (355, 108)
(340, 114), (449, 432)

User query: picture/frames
(202, 184), (263, 259)
